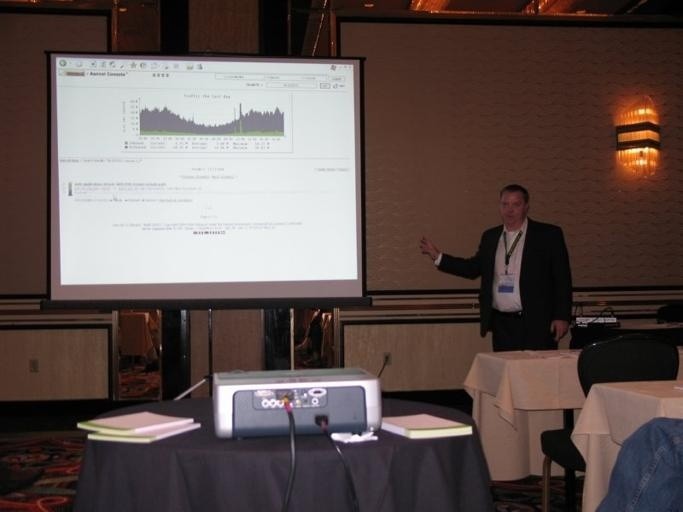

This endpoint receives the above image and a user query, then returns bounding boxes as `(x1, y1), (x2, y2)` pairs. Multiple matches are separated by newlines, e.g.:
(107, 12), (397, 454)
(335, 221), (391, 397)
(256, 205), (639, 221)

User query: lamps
(611, 94), (663, 182)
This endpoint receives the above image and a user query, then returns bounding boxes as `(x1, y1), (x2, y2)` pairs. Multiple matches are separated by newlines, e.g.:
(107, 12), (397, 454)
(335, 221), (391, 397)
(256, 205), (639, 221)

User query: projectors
(212, 367), (382, 438)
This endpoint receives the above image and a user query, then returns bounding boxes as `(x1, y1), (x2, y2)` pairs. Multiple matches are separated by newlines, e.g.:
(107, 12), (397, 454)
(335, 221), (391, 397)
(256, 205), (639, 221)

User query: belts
(489, 305), (525, 321)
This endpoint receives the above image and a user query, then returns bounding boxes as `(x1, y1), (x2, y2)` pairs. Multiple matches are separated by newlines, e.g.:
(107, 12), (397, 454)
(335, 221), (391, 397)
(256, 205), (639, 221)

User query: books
(381, 411), (473, 440)
(87, 421), (200, 444)
(76, 411), (195, 436)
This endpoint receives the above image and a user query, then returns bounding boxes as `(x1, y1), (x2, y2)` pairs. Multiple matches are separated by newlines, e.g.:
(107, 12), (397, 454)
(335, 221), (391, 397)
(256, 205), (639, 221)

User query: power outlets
(382, 351), (392, 367)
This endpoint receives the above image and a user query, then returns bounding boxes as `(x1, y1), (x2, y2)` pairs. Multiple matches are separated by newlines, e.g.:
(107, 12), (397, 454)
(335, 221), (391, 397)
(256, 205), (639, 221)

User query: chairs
(537, 338), (680, 511)
(654, 301), (682, 325)
(115, 310), (160, 377)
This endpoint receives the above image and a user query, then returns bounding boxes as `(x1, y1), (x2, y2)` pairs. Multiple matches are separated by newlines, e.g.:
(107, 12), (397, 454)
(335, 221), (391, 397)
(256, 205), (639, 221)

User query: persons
(421, 183), (572, 353)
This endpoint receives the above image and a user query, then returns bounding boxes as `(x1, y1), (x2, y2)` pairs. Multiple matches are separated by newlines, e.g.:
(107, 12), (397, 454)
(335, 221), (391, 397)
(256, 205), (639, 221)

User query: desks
(460, 346), (683, 483)
(76, 394), (496, 510)
(570, 378), (683, 511)
(571, 321), (682, 345)
